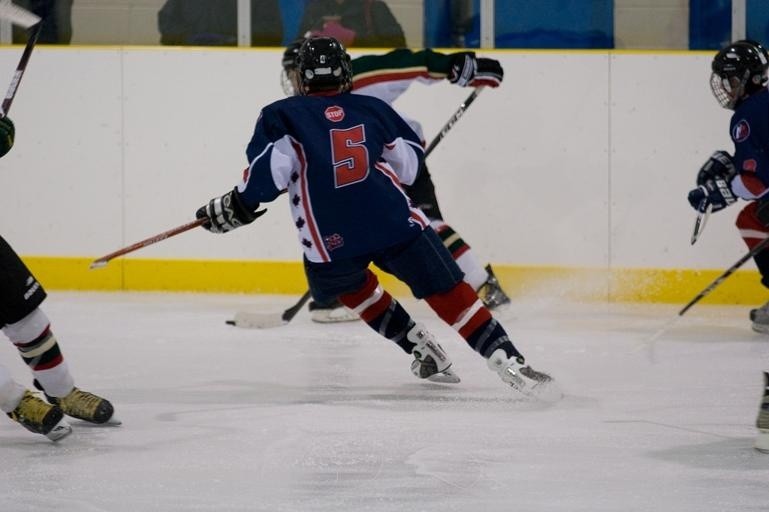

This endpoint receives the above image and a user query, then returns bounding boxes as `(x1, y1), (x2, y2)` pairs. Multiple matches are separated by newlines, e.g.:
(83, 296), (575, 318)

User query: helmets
(710, 39), (769, 109)
(277, 37), (353, 98)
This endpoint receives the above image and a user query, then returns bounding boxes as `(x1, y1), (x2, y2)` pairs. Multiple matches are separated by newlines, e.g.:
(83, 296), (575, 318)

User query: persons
(0, 114), (122, 444)
(278, 35), (511, 323)
(688, 39), (769, 454)
(196, 34), (564, 405)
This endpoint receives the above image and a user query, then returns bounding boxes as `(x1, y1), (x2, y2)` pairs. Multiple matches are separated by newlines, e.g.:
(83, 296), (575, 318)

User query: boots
(486, 348), (559, 396)
(406, 320), (453, 379)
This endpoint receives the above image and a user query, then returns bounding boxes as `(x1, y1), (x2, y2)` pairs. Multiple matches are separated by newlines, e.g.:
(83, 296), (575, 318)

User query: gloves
(197, 184), (267, 234)
(687, 149), (739, 215)
(450, 51), (504, 89)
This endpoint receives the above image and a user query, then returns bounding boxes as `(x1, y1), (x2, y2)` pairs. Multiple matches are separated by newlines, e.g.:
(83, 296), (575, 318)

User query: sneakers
(7, 389), (64, 435)
(43, 384), (115, 424)
(749, 302), (768, 322)
(476, 264), (499, 303)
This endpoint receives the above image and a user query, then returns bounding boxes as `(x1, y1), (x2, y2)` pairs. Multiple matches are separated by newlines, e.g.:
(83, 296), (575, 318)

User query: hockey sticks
(234, 86), (484, 330)
(1, 0), (43, 121)
(645, 235), (768, 361)
(89, 216), (211, 270)
(691, 196), (712, 243)
